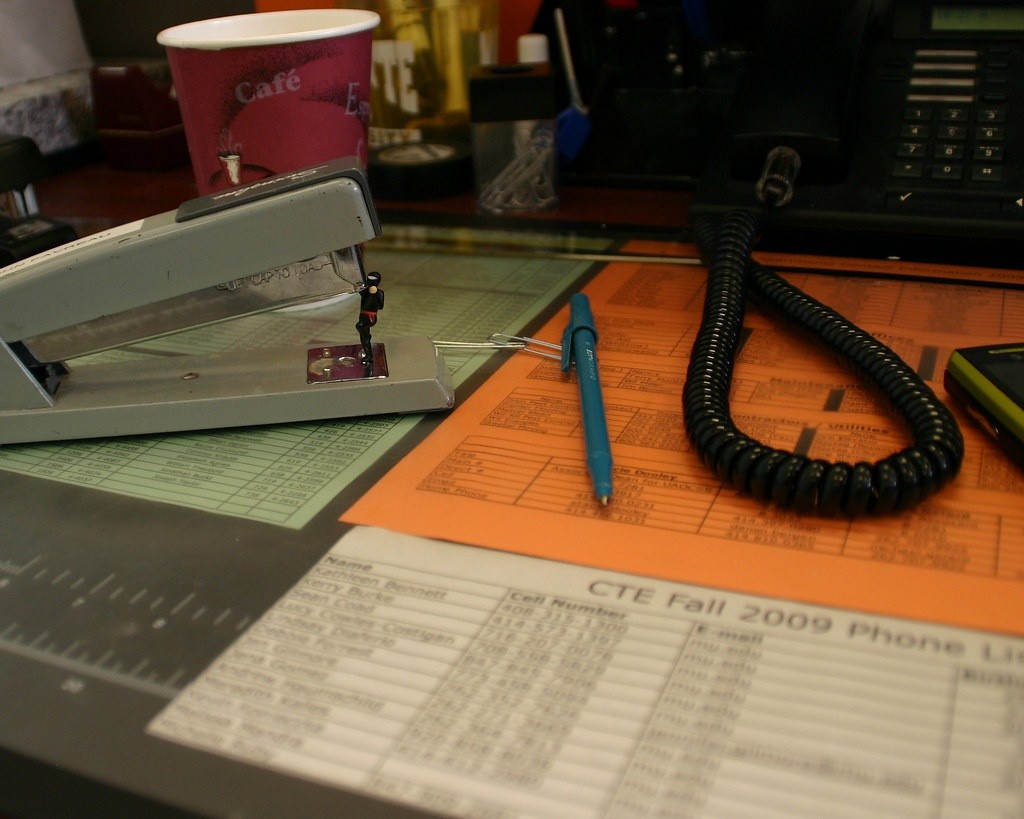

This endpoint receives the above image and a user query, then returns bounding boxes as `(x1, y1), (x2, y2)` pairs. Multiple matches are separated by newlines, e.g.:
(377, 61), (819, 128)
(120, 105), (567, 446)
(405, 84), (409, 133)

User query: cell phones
(944, 341), (1023, 470)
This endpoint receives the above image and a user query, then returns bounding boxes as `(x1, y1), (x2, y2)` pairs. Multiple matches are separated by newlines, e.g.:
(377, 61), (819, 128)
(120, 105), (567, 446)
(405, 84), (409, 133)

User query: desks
(0, 156), (1024, 819)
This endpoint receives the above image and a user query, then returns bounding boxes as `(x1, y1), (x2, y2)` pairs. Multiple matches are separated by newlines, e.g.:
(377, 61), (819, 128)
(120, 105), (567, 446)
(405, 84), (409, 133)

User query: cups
(157, 8), (380, 198)
(470, 61), (560, 218)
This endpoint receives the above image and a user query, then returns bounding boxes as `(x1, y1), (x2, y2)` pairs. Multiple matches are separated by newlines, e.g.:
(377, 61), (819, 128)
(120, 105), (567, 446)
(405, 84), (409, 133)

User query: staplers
(0, 160), (456, 449)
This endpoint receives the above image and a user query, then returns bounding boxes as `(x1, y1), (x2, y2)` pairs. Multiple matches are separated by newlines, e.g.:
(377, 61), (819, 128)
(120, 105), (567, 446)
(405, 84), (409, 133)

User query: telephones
(691, 29), (1024, 264)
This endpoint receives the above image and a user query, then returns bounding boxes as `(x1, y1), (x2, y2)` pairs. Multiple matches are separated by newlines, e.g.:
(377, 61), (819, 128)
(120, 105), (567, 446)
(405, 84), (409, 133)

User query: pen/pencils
(560, 292), (615, 509)
